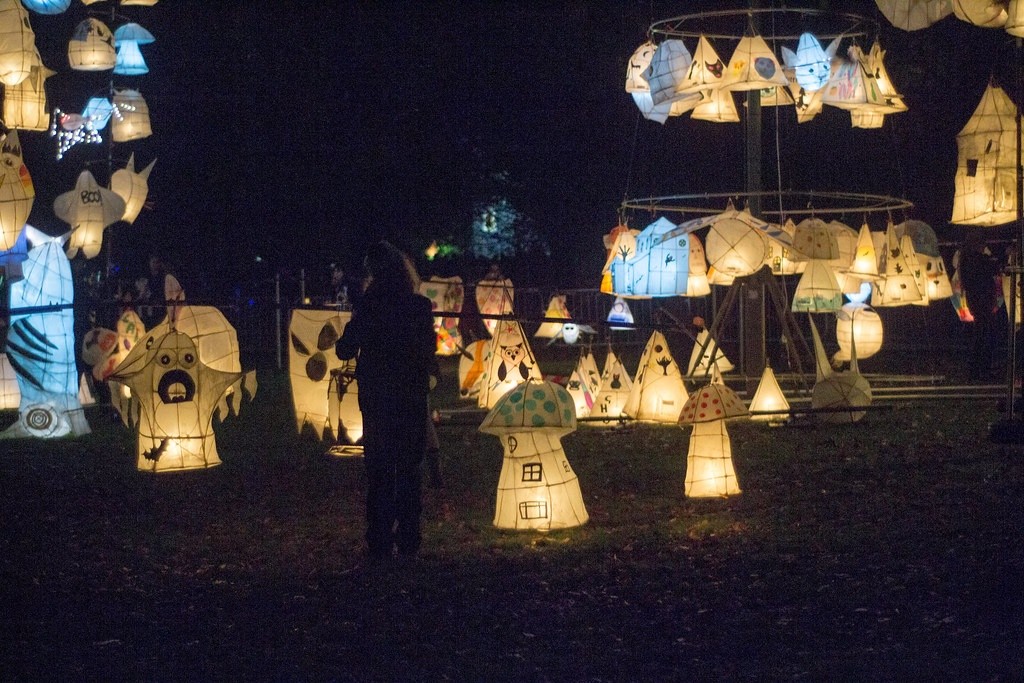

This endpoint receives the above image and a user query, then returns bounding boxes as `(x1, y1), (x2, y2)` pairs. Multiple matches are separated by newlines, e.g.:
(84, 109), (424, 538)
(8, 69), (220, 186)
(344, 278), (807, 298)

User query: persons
(335, 243), (435, 561)
(960, 230), (1005, 380)
